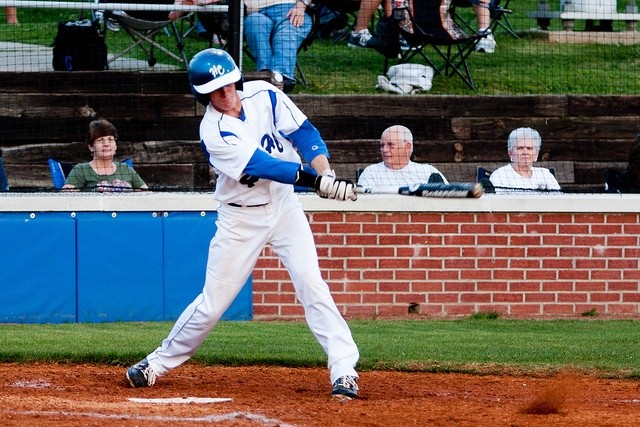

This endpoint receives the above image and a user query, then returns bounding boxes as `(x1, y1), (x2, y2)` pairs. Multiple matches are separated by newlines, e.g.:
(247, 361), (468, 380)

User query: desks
(527, 10), (640, 31)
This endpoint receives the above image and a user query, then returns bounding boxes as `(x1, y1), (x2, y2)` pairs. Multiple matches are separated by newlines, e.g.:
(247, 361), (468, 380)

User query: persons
(349, 0), (423, 51)
(241, 0), (314, 92)
(126, 48), (359, 400)
(0, 154), (9, 191)
(474, 0), (497, 53)
(169, 0), (241, 45)
(359, 125), (451, 192)
(63, 119), (148, 192)
(488, 127), (561, 193)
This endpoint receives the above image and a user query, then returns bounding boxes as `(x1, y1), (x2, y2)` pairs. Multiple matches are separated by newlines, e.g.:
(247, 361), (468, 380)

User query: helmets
(187, 48), (243, 107)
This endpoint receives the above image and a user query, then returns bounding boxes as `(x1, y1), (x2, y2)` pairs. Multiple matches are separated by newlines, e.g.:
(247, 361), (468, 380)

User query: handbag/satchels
(374, 63), (433, 94)
(375, 8), (425, 58)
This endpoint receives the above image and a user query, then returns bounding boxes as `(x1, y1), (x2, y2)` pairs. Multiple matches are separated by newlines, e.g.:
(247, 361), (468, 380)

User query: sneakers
(332, 375), (360, 401)
(476, 28), (497, 53)
(398, 34), (421, 52)
(347, 28), (376, 52)
(126, 358), (158, 388)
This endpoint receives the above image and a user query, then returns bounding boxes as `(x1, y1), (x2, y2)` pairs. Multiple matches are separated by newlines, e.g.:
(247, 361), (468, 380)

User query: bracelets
(299, 0), (309, 6)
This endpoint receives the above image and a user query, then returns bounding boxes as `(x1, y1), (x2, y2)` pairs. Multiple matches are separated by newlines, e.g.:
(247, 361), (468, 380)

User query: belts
(228, 202), (269, 209)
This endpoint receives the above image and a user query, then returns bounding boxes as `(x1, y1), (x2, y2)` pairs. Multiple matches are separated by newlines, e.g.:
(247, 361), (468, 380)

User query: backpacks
(51, 17), (108, 71)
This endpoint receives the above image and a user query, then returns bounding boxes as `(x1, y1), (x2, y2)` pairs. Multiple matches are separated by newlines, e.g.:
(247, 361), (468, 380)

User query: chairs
(218, 3), (320, 86)
(197, 2), (258, 64)
(48, 158), (132, 191)
(91, 0), (191, 71)
(356, 167), (364, 181)
(392, 7), (491, 91)
(452, 0), (520, 40)
(475, 165), (557, 184)
(325, 5), (382, 43)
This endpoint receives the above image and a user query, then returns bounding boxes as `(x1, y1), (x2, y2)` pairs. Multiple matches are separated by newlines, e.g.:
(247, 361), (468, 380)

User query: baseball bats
(352, 182), (483, 198)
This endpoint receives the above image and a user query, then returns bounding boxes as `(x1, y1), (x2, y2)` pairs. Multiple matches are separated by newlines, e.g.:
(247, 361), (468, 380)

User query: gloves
(315, 168), (358, 200)
